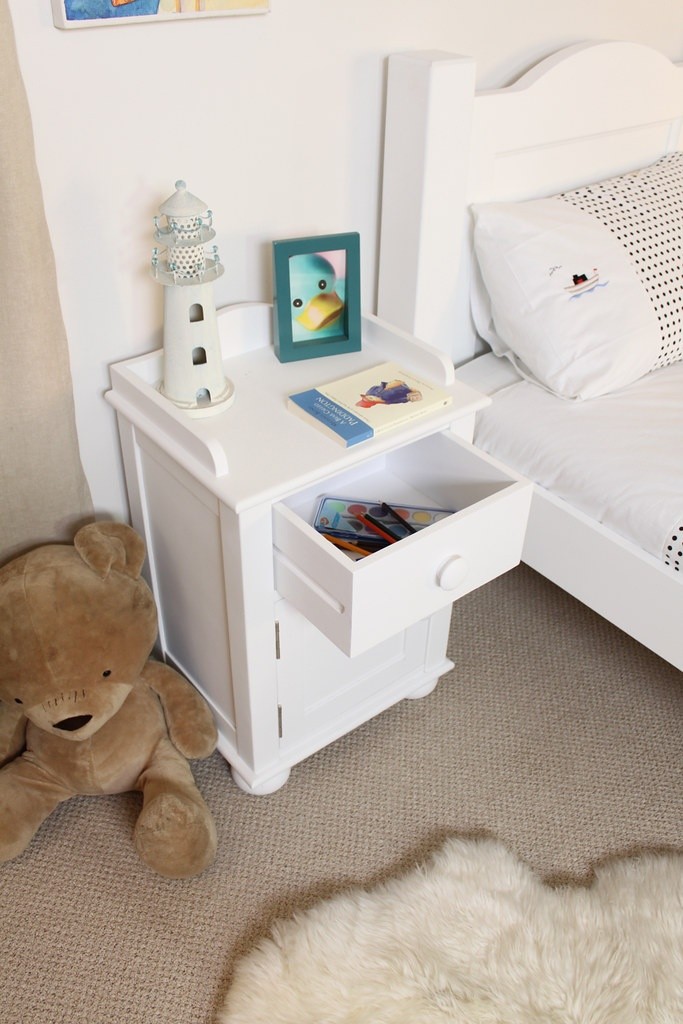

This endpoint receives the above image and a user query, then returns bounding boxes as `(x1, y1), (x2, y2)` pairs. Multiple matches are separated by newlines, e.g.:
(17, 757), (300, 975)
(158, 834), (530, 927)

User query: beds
(371, 37), (683, 675)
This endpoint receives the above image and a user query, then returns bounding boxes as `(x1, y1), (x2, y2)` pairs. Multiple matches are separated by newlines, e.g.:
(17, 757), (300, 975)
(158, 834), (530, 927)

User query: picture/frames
(51, 1), (270, 29)
(272, 232), (361, 364)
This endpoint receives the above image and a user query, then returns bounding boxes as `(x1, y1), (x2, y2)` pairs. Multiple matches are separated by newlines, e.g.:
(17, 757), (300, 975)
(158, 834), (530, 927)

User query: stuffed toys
(0, 519), (221, 876)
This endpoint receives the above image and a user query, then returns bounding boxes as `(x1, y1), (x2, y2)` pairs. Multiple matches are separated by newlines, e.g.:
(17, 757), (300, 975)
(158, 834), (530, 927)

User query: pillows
(466, 151), (683, 402)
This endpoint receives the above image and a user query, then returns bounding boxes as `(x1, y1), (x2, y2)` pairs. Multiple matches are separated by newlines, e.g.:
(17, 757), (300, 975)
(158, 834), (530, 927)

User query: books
(284, 359), (454, 450)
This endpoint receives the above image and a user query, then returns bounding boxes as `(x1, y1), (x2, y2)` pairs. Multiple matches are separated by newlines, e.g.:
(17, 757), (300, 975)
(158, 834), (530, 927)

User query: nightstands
(102, 288), (532, 796)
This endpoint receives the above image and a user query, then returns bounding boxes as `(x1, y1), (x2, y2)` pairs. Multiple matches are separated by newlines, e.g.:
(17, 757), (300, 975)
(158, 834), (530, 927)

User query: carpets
(212, 825), (683, 1023)
